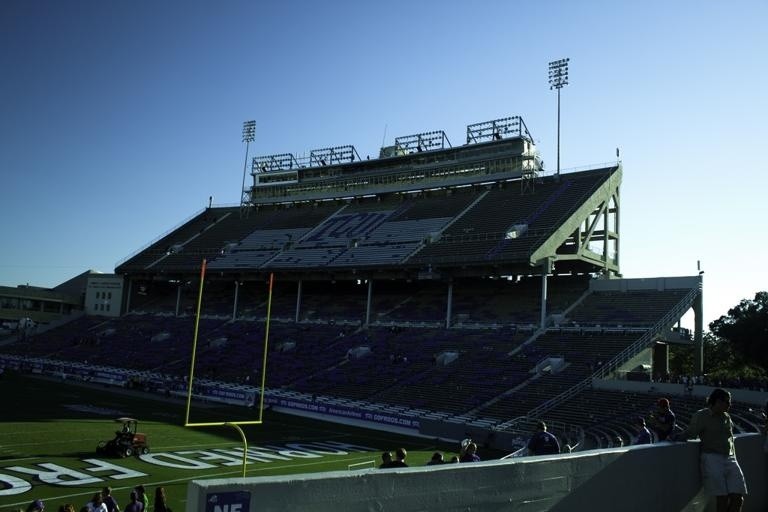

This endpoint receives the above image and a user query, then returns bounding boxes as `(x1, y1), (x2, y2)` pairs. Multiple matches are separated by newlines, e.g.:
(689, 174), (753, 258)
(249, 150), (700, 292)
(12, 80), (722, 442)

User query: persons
(527, 419), (561, 455)
(384, 446), (408, 469)
(121, 422), (130, 433)
(654, 397), (676, 440)
(8, 484), (173, 511)
(614, 436), (624, 447)
(666, 387), (749, 512)
(460, 443), (481, 462)
(563, 444), (571, 453)
(650, 372), (768, 397)
(427, 451), (446, 465)
(451, 455), (461, 463)
(632, 415), (651, 446)
(378, 451), (395, 468)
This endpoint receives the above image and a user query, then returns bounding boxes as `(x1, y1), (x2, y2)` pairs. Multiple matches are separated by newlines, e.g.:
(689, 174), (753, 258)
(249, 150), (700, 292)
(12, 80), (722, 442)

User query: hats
(655, 399), (669, 406)
(396, 447), (407, 459)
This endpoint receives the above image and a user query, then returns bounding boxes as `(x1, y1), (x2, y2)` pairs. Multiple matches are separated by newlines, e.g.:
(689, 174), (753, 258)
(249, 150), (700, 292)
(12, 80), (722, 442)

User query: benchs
(582, 397), (767, 457)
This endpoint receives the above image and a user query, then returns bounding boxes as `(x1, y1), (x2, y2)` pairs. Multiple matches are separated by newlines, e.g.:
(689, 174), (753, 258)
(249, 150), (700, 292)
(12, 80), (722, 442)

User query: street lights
(237, 118), (258, 207)
(547, 56), (569, 176)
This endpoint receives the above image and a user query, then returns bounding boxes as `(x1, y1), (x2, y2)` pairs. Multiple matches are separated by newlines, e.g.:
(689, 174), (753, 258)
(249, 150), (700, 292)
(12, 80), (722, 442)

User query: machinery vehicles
(95, 417), (150, 456)
